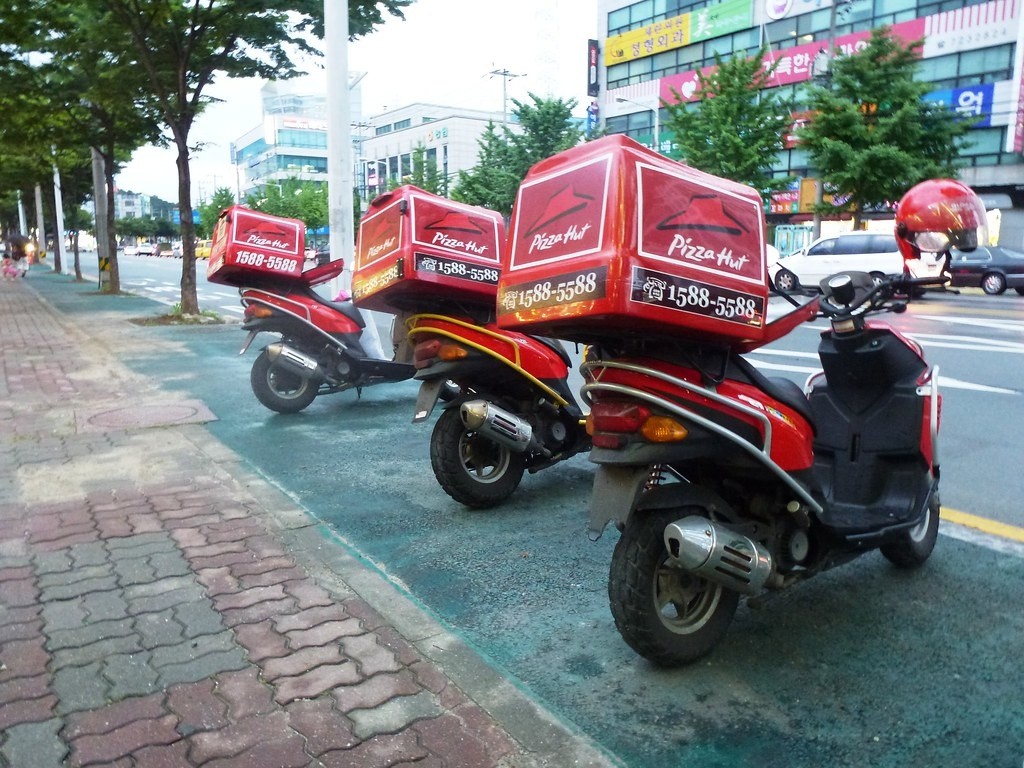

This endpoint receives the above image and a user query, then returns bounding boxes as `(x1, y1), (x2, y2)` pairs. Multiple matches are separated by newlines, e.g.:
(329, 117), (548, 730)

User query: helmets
(894, 179), (988, 259)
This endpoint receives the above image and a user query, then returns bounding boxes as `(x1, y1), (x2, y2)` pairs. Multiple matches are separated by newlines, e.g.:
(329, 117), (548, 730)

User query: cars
(303, 241), (330, 265)
(947, 243), (1024, 296)
(117, 237), (212, 261)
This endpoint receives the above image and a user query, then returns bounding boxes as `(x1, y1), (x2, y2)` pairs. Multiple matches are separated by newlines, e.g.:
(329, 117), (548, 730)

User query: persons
(0, 254), (15, 282)
(7, 229), (30, 277)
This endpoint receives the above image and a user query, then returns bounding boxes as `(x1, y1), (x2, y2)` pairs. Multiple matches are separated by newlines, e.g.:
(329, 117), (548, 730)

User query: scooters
(491, 133), (990, 664)
(207, 205), (418, 415)
(350, 185), (598, 511)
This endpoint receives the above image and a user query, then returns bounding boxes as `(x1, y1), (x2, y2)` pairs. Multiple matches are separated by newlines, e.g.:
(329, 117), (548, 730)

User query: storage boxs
(351, 184), (505, 316)
(206, 204), (344, 285)
(495, 134), (821, 352)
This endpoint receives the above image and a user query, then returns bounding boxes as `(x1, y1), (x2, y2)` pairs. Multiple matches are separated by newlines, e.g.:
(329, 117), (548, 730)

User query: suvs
(766, 229), (952, 297)
